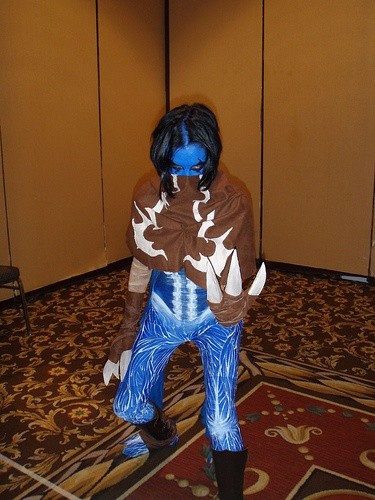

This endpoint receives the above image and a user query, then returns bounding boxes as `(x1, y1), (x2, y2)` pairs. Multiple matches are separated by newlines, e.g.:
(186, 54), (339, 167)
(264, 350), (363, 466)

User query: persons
(102, 102), (266, 499)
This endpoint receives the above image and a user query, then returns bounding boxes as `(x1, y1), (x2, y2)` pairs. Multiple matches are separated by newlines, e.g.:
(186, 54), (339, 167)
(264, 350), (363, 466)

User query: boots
(122, 399), (178, 458)
(211, 447), (247, 500)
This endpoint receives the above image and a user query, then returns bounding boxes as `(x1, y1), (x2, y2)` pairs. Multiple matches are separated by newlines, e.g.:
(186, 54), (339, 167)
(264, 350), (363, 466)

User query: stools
(0, 265), (31, 333)
